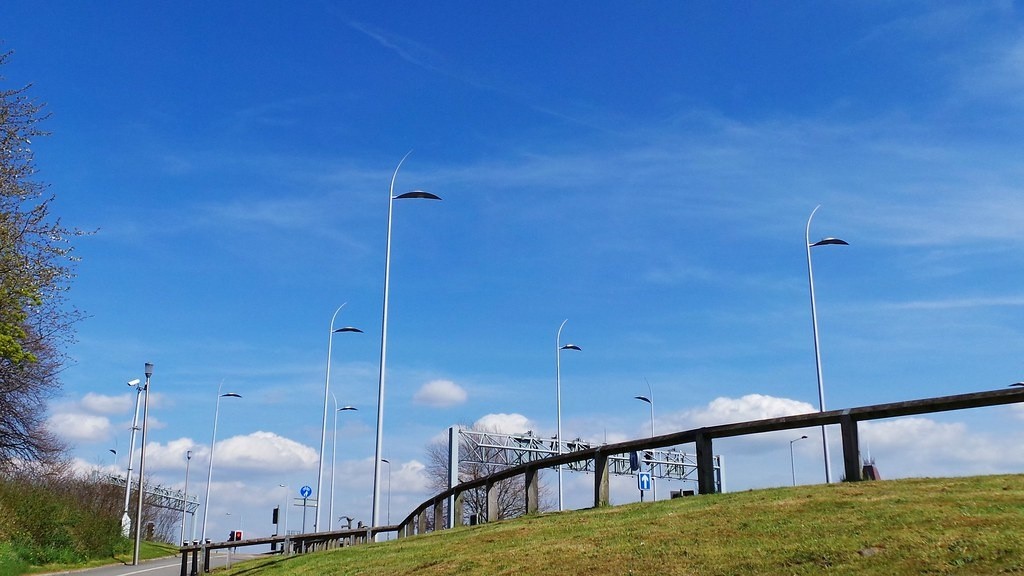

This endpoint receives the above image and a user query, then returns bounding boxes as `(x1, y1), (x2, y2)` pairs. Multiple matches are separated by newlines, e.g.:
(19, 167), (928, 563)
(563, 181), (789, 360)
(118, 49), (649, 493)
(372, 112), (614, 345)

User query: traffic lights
(273, 506), (280, 524)
(236, 531), (242, 541)
(229, 531), (235, 541)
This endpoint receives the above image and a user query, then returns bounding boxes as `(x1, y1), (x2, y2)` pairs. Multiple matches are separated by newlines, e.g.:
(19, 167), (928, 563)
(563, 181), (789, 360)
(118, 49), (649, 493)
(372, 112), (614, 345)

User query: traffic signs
(638, 473), (651, 491)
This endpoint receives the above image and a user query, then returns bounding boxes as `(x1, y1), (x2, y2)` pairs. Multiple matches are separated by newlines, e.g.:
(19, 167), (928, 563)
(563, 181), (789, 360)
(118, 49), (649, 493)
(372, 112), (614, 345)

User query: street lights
(201, 379), (242, 544)
(368, 146), (442, 542)
(131, 361), (153, 566)
(556, 318), (582, 512)
(804, 203), (851, 485)
(790, 436), (808, 487)
(328, 393), (357, 532)
(634, 377), (657, 501)
(314, 301), (365, 536)
